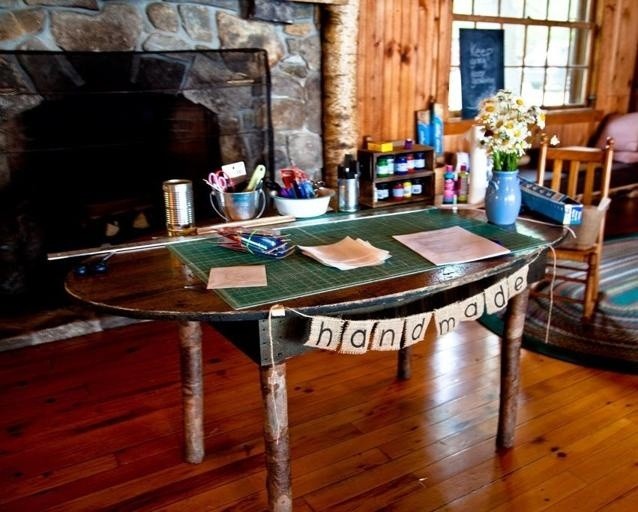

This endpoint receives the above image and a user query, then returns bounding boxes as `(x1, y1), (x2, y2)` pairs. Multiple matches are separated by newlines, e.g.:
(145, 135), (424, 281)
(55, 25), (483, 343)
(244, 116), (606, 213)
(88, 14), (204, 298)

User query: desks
(63, 205), (567, 512)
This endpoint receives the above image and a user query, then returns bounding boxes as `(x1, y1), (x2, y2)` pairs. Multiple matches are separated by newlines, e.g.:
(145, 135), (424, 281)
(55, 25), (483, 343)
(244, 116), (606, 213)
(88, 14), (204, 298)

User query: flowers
(473, 88), (559, 169)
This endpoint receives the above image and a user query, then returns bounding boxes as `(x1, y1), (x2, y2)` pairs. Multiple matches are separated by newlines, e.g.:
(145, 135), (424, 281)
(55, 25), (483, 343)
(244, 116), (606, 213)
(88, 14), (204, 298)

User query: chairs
(530, 132), (615, 324)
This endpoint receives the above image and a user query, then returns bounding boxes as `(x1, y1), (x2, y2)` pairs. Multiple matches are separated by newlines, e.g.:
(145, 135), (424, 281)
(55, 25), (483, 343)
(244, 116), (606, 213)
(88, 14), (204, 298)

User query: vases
(484, 171), (522, 224)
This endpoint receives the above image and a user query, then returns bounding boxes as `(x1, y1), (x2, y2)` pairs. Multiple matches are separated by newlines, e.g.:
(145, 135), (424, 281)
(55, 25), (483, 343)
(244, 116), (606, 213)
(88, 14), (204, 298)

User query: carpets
(474, 234), (638, 377)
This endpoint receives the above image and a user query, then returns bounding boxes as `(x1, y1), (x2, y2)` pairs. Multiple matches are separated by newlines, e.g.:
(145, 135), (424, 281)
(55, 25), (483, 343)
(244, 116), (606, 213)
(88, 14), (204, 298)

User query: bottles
(338, 155), (359, 213)
(443, 164), (469, 203)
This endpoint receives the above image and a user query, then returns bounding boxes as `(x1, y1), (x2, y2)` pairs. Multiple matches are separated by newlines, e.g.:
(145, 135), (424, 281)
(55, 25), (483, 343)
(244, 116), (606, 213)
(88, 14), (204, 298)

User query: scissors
(209, 170), (236, 193)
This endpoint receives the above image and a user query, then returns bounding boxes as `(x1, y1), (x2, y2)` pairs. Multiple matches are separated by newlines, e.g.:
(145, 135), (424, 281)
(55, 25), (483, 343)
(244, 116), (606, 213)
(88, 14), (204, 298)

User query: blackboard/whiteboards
(460, 29), (505, 119)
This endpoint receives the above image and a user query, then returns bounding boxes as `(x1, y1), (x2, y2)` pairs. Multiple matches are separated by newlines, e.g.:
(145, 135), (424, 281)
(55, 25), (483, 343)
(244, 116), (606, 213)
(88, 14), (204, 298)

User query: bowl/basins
(267, 186), (337, 220)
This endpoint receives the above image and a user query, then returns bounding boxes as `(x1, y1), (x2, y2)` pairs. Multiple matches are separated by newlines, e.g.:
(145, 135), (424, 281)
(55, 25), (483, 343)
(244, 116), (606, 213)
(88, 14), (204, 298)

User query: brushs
(232, 164), (266, 220)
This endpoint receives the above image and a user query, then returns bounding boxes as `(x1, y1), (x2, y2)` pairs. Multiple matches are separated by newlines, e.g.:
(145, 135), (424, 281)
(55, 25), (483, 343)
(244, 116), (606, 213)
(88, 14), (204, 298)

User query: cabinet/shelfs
(357, 142), (436, 210)
